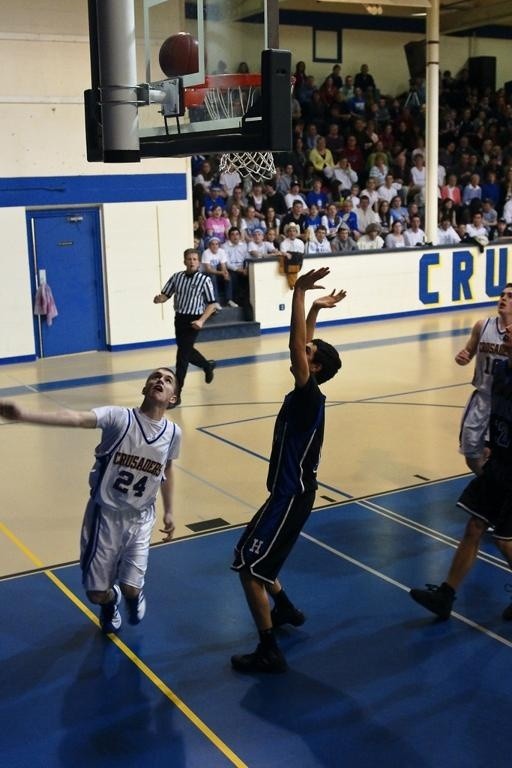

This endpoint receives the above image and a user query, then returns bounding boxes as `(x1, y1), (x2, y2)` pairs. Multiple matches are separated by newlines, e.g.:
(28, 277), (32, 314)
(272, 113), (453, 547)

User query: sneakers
(123, 590), (148, 626)
(215, 300), (240, 311)
(229, 641), (289, 675)
(98, 583), (124, 634)
(408, 583), (454, 621)
(269, 603), (306, 630)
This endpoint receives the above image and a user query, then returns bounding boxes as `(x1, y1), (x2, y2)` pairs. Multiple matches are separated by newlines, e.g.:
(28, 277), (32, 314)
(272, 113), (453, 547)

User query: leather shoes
(167, 396), (182, 410)
(205, 359), (217, 384)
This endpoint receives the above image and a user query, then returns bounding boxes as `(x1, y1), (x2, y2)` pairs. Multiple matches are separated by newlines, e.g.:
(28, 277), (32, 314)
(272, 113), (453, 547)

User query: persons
(410, 324), (512, 620)
(190, 60), (305, 309)
(231, 267), (348, 673)
(438, 70), (511, 245)
(279, 61), (426, 256)
(455, 282), (512, 475)
(153, 247), (218, 409)
(0, 366), (183, 633)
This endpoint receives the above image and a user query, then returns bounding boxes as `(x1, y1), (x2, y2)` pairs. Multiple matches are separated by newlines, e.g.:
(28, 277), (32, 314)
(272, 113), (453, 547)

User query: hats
(283, 221), (301, 237)
(290, 180), (300, 186)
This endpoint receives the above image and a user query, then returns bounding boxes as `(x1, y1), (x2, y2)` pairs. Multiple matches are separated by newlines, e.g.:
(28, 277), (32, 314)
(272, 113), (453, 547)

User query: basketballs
(159, 32), (208, 78)
